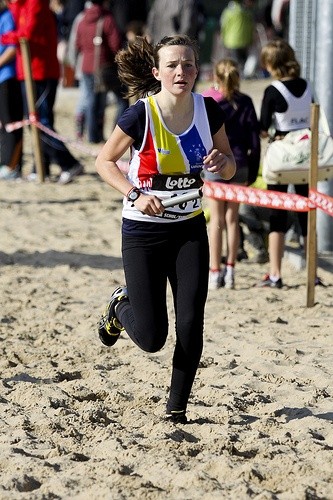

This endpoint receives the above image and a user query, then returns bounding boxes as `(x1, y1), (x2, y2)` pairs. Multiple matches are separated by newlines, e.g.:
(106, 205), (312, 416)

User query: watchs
(127, 186), (142, 207)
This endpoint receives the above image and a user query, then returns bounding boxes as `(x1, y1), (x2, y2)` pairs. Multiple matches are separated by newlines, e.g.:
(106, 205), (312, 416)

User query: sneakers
(222, 250), (248, 265)
(222, 276), (234, 289)
(59, 164), (83, 185)
(256, 276), (283, 289)
(98, 284), (128, 347)
(0, 165), (18, 180)
(26, 175), (49, 183)
(208, 273), (222, 289)
(160, 410), (187, 424)
(254, 252), (269, 263)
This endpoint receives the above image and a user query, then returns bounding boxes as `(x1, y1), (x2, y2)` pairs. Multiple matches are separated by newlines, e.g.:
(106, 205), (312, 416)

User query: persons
(260, 40), (326, 289)
(50, 0), (199, 146)
(95, 34), (236, 425)
(0, 0), (25, 179)
(220, 0), (253, 80)
(0, 0), (83, 184)
(207, 59), (261, 289)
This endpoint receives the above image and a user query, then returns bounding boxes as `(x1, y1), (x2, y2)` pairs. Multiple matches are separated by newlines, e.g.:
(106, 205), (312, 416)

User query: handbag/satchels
(95, 67), (118, 94)
(262, 106), (333, 185)
